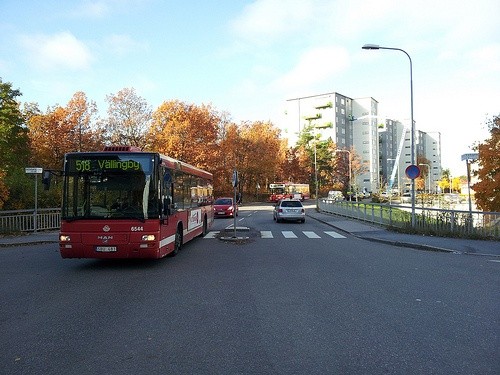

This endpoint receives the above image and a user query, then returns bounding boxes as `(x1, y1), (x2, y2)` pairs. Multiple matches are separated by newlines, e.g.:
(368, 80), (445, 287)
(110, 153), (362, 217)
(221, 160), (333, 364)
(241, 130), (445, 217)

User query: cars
(213, 197), (238, 218)
(335, 193), (360, 202)
(272, 199), (306, 223)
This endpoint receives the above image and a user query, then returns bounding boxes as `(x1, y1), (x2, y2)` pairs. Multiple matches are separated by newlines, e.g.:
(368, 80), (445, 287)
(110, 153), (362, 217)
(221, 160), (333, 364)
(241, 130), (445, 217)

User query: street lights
(419, 164), (431, 195)
(386, 158), (401, 196)
(334, 149), (352, 202)
(361, 43), (417, 230)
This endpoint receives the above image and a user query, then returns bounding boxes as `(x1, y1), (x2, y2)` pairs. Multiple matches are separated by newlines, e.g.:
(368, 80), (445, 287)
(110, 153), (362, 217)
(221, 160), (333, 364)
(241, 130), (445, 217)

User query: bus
(268, 181), (310, 203)
(41, 146), (216, 260)
